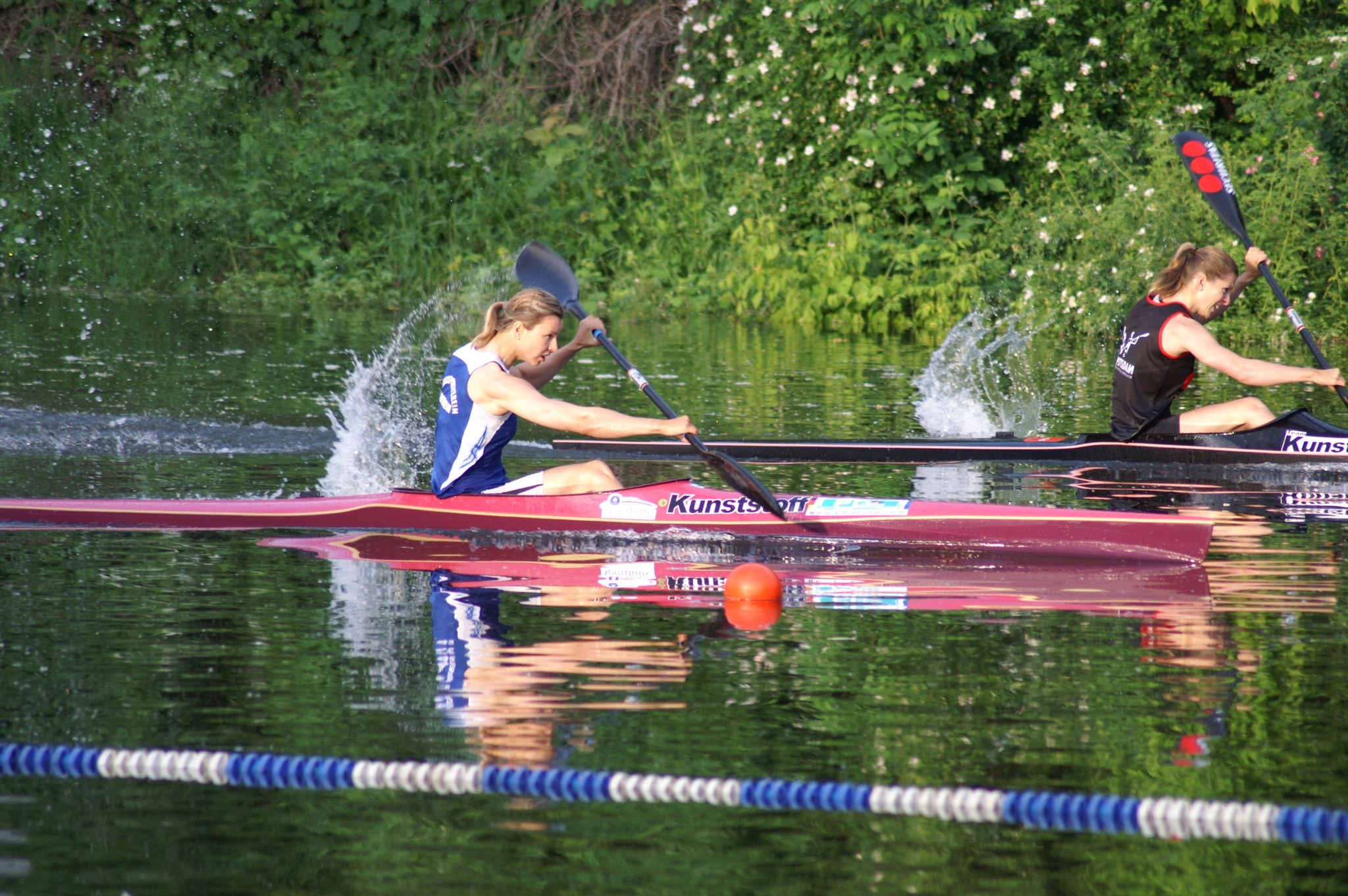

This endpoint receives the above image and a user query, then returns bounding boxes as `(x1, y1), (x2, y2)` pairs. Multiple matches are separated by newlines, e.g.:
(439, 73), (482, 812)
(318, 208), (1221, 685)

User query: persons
(430, 287), (697, 496)
(1109, 241), (1346, 438)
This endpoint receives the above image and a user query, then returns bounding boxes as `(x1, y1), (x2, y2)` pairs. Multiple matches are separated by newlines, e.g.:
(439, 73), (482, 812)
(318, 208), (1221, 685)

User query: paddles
(515, 240), (788, 522)
(1174, 130), (1348, 410)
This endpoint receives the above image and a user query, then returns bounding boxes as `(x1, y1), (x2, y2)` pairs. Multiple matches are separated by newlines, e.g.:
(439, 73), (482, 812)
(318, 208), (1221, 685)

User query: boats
(0, 476), (1218, 570)
(551, 427), (1348, 463)
